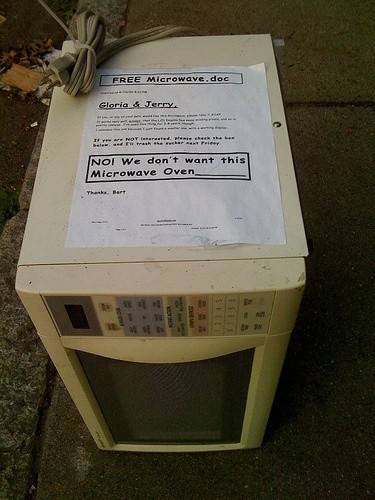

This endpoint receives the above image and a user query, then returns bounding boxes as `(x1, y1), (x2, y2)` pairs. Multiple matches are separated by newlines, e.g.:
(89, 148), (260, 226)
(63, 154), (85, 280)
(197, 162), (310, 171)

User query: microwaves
(15, 32), (308, 454)
